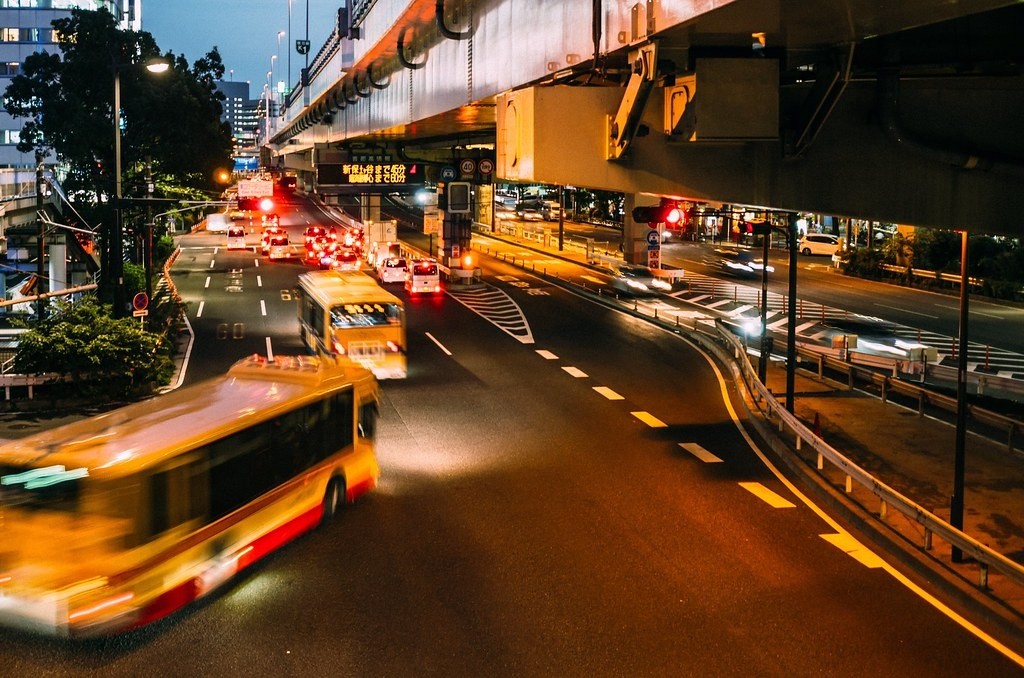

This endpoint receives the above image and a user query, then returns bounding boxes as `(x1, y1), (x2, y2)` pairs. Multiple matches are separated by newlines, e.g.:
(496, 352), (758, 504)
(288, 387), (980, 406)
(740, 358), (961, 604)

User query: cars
(262, 214), (280, 229)
(226, 225), (248, 250)
(377, 257), (409, 285)
(303, 226), (364, 270)
(610, 267), (661, 297)
(799, 233), (853, 255)
(522, 209), (542, 221)
(403, 258), (440, 294)
(260, 228), (291, 261)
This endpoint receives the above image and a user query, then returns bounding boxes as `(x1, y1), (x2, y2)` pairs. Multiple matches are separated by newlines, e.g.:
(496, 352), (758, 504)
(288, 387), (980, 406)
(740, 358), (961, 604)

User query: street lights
(270, 55), (276, 127)
(278, 32), (286, 117)
(257, 72), (272, 125)
(114, 57), (171, 198)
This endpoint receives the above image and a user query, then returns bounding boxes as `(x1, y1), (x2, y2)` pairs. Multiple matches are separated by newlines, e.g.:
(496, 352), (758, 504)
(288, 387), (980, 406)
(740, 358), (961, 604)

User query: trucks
(207, 214), (236, 235)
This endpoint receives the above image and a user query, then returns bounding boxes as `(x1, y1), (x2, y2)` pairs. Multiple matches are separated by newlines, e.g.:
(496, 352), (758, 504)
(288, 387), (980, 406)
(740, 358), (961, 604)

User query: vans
(542, 203), (566, 221)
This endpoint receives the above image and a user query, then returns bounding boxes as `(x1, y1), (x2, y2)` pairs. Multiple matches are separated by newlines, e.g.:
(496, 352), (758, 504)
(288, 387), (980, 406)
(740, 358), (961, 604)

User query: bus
(295, 268), (409, 380)
(0, 355), (381, 641)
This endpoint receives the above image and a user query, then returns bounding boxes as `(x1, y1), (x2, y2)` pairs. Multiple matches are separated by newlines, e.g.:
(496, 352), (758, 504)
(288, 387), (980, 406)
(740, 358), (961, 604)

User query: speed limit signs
(461, 159), (477, 175)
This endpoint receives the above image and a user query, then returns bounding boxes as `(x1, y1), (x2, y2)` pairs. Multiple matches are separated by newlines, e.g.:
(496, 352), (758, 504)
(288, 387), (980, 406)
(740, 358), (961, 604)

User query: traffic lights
(633, 206), (684, 225)
(737, 220), (767, 235)
